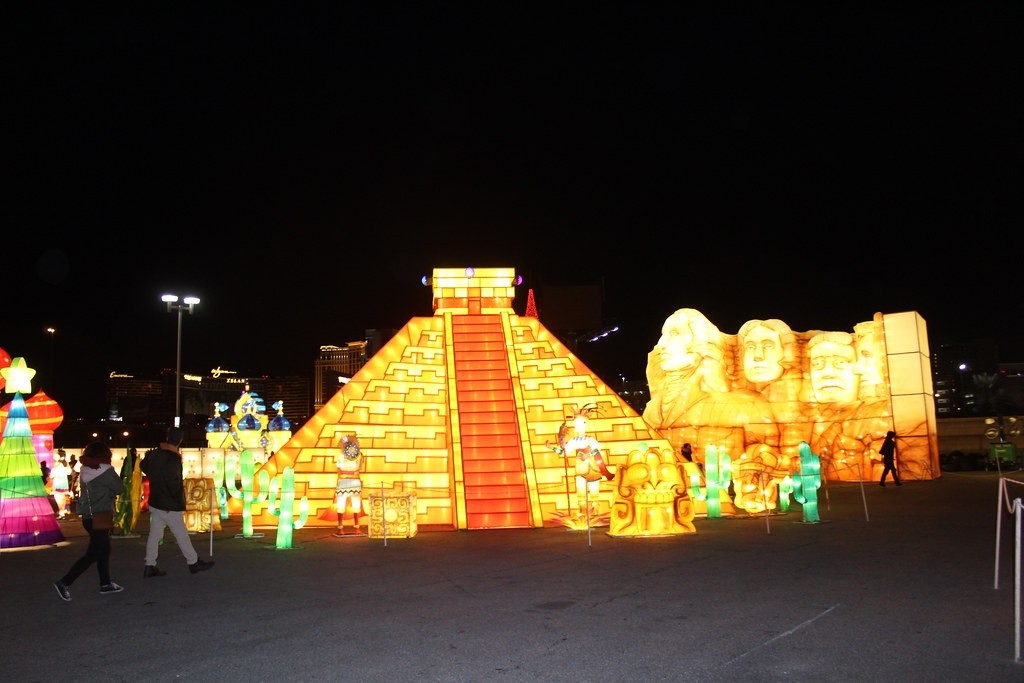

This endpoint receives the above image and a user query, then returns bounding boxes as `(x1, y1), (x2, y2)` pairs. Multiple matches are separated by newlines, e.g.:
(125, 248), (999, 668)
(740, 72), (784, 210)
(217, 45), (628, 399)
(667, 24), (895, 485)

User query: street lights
(575, 324), (620, 360)
(162, 295), (200, 416)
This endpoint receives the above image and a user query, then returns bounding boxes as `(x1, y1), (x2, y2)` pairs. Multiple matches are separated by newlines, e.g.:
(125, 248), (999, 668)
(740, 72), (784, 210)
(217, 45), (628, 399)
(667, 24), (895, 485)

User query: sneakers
(100, 583), (125, 595)
(54, 580), (73, 603)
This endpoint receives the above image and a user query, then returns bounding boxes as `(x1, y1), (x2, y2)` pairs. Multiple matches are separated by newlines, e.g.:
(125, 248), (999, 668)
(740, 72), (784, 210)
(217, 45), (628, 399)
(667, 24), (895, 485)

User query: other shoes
(878, 483), (886, 488)
(56, 512), (67, 519)
(190, 560), (215, 572)
(144, 566), (168, 577)
(896, 483), (904, 487)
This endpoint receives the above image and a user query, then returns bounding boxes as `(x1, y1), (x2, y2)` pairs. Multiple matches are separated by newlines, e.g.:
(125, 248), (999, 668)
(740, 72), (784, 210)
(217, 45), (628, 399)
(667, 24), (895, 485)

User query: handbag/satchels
(91, 511), (114, 531)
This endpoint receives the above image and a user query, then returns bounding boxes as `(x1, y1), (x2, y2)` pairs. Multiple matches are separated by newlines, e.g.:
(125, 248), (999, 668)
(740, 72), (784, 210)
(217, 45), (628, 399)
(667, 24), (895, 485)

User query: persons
(544, 413), (616, 520)
(138, 427), (216, 578)
(641, 307), (895, 483)
(65, 453), (79, 492)
(334, 435), (367, 535)
(878, 431), (906, 488)
(40, 461), (61, 520)
(52, 440), (126, 601)
(121, 447), (138, 480)
